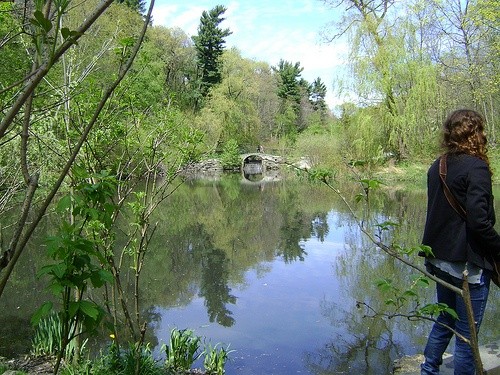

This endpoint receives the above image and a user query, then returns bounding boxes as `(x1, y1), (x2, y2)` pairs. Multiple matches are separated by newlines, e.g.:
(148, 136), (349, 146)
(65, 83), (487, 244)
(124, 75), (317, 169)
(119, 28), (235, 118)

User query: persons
(419, 110), (500, 375)
(256, 145), (264, 153)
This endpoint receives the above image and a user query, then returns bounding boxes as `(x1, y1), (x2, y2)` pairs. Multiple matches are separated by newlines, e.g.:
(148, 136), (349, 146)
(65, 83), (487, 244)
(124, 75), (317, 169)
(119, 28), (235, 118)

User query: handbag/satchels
(487, 252), (500, 289)
